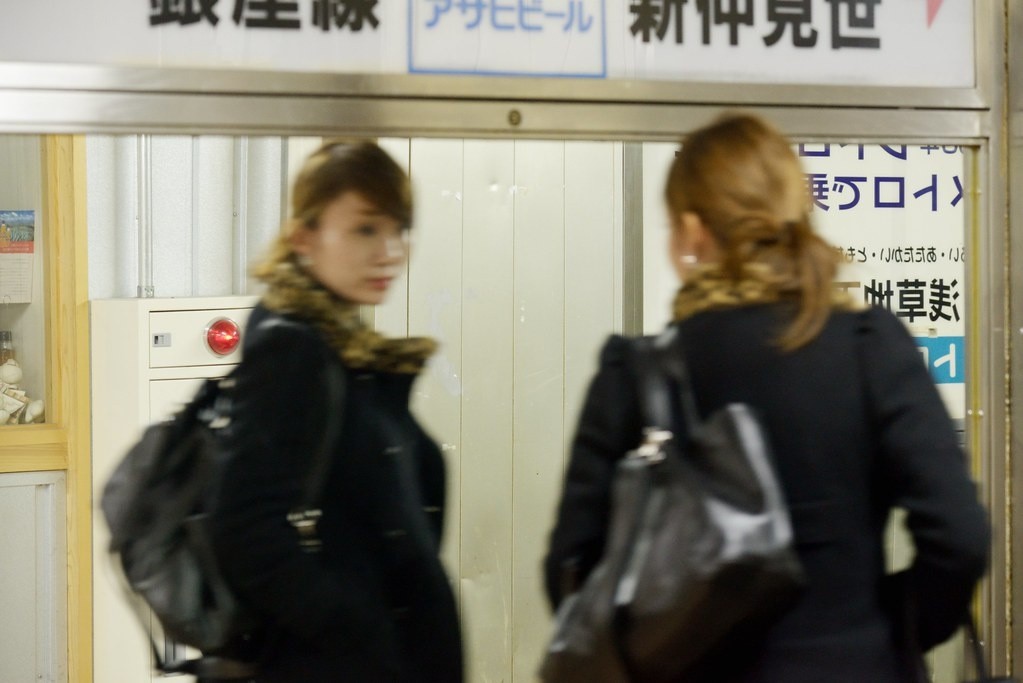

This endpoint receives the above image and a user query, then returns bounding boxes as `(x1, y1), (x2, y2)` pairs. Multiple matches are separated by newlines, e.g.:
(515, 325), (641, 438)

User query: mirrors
(0, 133), (988, 682)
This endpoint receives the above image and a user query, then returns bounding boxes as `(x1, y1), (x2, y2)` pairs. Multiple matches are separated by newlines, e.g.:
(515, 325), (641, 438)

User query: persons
(535, 113), (989, 683)
(95, 141), (465, 683)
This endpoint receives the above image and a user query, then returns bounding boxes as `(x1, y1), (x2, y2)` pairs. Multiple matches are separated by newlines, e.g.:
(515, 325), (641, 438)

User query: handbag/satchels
(538, 336), (807, 683)
(96, 319), (345, 675)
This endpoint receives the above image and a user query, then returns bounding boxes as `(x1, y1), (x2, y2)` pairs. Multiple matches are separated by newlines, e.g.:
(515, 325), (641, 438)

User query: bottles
(1, 330), (13, 368)
